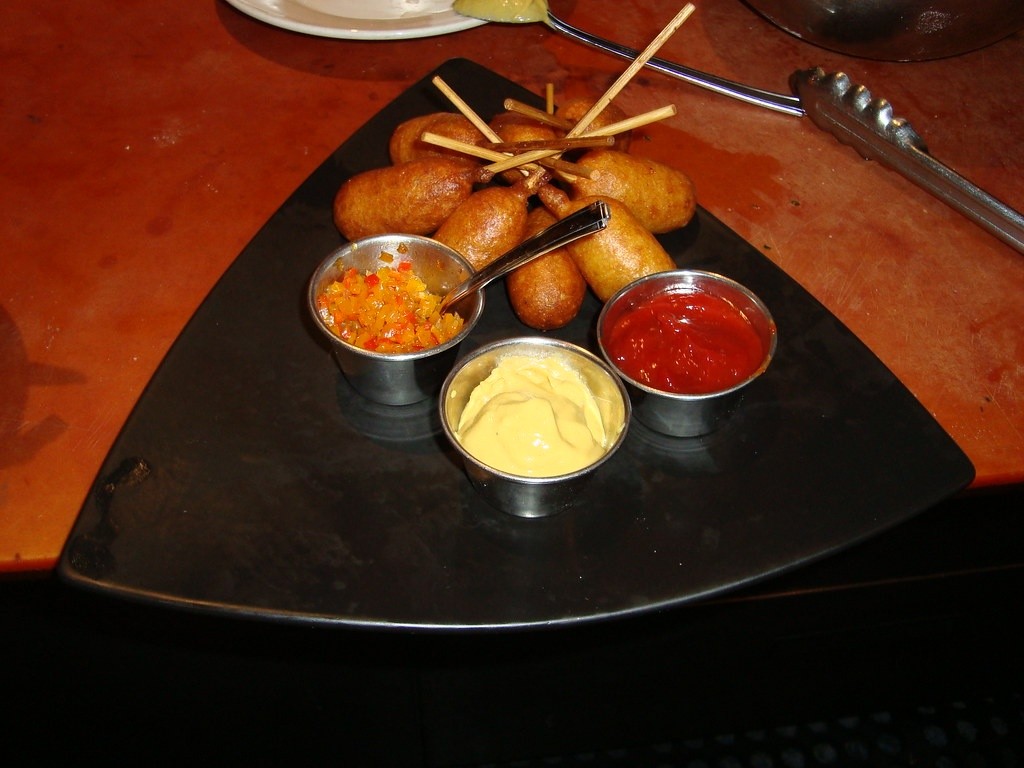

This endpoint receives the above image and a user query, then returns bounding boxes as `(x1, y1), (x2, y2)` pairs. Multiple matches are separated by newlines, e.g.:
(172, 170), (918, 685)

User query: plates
(58, 58), (974, 632)
(227, 0), (491, 39)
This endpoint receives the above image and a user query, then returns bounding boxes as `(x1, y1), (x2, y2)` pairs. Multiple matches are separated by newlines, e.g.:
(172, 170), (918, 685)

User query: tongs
(796, 67), (1024, 256)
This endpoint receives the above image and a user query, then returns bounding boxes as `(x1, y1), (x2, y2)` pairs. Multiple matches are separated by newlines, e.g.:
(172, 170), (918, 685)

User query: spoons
(451, 0), (808, 117)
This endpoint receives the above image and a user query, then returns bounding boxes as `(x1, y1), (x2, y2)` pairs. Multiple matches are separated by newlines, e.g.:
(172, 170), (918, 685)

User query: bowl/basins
(307, 234), (486, 406)
(596, 270), (777, 437)
(438, 337), (631, 518)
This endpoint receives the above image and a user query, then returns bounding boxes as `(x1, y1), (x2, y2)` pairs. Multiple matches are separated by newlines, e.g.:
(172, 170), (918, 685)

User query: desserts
(460, 355), (607, 478)
